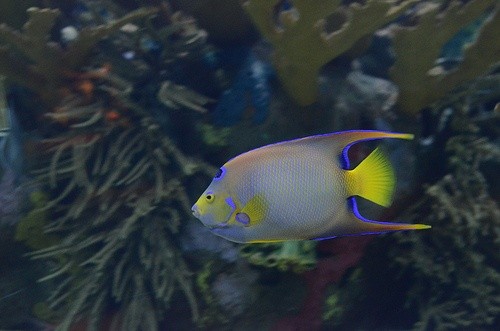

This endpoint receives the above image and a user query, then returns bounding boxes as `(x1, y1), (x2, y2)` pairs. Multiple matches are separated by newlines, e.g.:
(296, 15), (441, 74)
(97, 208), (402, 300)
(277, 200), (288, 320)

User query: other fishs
(191, 129), (432, 244)
